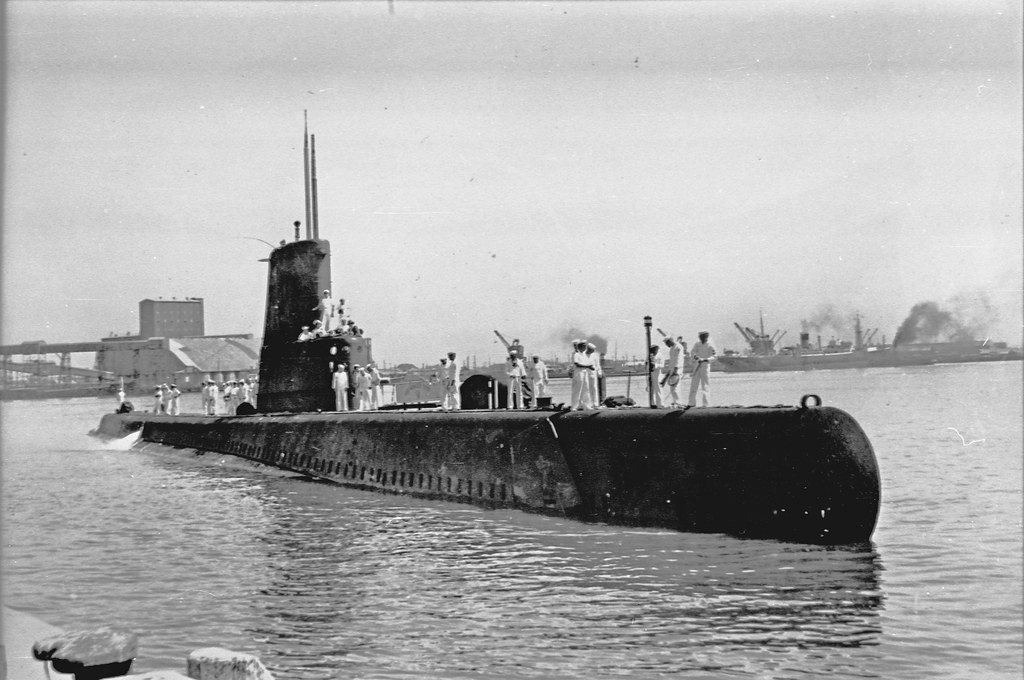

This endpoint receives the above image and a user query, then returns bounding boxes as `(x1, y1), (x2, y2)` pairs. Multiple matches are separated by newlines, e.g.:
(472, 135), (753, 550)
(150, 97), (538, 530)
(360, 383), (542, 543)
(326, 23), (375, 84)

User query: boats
(460, 309), (1024, 378)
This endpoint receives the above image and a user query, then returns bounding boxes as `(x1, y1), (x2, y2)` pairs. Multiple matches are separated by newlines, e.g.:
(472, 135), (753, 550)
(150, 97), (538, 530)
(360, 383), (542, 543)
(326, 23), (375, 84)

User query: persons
(222, 379), (248, 415)
(332, 364), (350, 411)
(662, 335), (684, 405)
(570, 339), (602, 410)
(116, 389), (125, 403)
(438, 352), (461, 411)
(645, 345), (664, 409)
(686, 331), (716, 407)
(313, 289), (350, 333)
(298, 320), (323, 340)
(154, 384), (181, 415)
(201, 380), (218, 415)
(366, 363), (380, 409)
(529, 355), (549, 407)
(352, 363), (371, 411)
(504, 350), (527, 409)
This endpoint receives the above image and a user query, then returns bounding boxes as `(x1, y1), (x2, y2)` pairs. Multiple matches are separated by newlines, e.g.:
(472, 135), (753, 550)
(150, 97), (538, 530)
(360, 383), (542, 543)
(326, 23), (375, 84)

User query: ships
(84, 106), (884, 547)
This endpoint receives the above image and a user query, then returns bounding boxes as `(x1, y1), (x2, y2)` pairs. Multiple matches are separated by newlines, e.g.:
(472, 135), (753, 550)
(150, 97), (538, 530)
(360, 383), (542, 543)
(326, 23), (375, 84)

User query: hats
(336, 364), (371, 373)
(509, 350), (518, 355)
(447, 352), (456, 356)
(323, 290), (330, 294)
(201, 380), (215, 385)
(697, 331), (708, 337)
(662, 335), (671, 343)
(222, 379), (244, 386)
(578, 340), (587, 345)
(588, 343), (597, 350)
(572, 339), (581, 345)
(533, 355), (539, 359)
